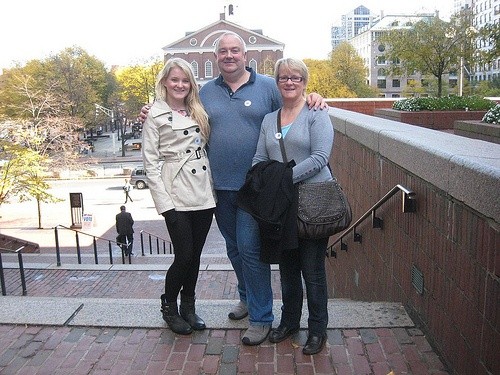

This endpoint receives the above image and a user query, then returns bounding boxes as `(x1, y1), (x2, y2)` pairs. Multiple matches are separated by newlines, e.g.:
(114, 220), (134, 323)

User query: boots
(159, 293), (192, 335)
(179, 289), (206, 330)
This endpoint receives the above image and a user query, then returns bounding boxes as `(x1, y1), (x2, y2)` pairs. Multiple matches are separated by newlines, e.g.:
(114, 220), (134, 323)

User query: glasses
(276, 73), (305, 82)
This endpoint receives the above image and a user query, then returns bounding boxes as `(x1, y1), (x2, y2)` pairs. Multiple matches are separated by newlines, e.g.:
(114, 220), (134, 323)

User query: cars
(129, 166), (149, 189)
(79, 139), (95, 154)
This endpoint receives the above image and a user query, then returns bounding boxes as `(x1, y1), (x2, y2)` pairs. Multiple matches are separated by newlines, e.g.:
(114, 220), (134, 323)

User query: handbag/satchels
(294, 177), (352, 241)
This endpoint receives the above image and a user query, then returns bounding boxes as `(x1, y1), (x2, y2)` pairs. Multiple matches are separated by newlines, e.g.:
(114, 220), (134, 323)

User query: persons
(251, 58), (334, 354)
(141, 31), (330, 345)
(116, 206), (134, 256)
(142, 58), (216, 335)
(123, 178), (133, 204)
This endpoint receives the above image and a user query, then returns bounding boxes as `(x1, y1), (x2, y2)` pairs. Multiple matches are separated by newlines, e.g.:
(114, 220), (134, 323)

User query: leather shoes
(301, 330), (329, 355)
(268, 319), (301, 344)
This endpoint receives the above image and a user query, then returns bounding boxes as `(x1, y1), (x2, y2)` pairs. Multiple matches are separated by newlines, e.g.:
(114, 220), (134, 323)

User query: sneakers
(240, 324), (273, 346)
(227, 301), (248, 320)
